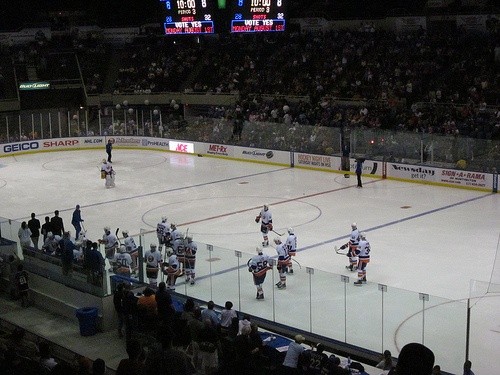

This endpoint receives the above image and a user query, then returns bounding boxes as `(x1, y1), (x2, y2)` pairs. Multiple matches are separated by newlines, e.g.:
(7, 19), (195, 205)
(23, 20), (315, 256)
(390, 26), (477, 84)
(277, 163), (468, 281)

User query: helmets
(256, 246), (262, 252)
(186, 235), (192, 239)
(102, 158), (106, 163)
(47, 232), (53, 237)
(288, 228), (294, 234)
(273, 236), (282, 244)
(352, 223), (356, 230)
(166, 248), (173, 253)
(122, 230), (128, 234)
(161, 216), (168, 221)
(360, 233), (365, 238)
(104, 227), (110, 232)
(264, 205), (269, 209)
(150, 243), (156, 247)
(120, 245), (126, 252)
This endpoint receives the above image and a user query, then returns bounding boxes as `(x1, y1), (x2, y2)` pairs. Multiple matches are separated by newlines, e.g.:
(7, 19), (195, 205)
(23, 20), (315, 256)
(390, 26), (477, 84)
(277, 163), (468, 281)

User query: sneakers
(166, 276), (195, 289)
(276, 281), (286, 288)
(346, 266), (353, 272)
(354, 278), (366, 285)
(108, 267), (113, 272)
(131, 272), (136, 276)
(288, 269), (294, 274)
(256, 292), (264, 300)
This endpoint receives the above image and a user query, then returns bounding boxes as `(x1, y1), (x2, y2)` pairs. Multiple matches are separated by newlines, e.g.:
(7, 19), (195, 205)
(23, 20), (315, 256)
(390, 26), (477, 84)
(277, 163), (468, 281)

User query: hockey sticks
(258, 221), (286, 236)
(269, 244), (302, 270)
(115, 227), (121, 244)
(334, 245), (347, 256)
(81, 222), (87, 233)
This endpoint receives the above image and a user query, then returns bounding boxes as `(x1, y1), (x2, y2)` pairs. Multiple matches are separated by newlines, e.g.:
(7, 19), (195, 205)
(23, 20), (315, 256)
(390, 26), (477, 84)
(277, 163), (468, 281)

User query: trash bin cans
(75, 307), (98, 336)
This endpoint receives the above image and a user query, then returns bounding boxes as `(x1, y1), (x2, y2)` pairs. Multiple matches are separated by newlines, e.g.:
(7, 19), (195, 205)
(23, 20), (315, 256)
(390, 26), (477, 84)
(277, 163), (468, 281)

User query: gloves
(269, 223), (272, 230)
(256, 216), (260, 222)
(340, 244), (347, 249)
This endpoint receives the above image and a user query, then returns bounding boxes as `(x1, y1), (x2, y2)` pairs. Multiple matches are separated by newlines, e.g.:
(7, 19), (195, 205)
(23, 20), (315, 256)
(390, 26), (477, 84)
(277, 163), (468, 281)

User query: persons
(340, 222), (361, 272)
(113, 322), (441, 375)
(98, 227), (138, 278)
(18, 210), (105, 267)
(15, 265), (29, 308)
(157, 217), (197, 285)
(101, 159), (116, 188)
(72, 205), (84, 241)
(162, 247), (180, 292)
(255, 203), (273, 247)
(286, 227), (296, 275)
(274, 236), (289, 289)
(347, 232), (370, 286)
(114, 280), (251, 348)
(106, 139), (112, 163)
(248, 246), (273, 299)
(143, 242), (163, 287)
(0, 14), (500, 172)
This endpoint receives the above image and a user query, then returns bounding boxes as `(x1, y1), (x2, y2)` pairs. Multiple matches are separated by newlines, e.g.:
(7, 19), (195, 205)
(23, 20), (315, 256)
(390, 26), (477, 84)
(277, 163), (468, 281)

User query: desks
(134, 284), (391, 375)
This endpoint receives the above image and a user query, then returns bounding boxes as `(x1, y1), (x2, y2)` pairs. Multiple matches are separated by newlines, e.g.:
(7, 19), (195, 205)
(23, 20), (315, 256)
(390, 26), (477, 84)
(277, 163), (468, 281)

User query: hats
(295, 335), (306, 341)
